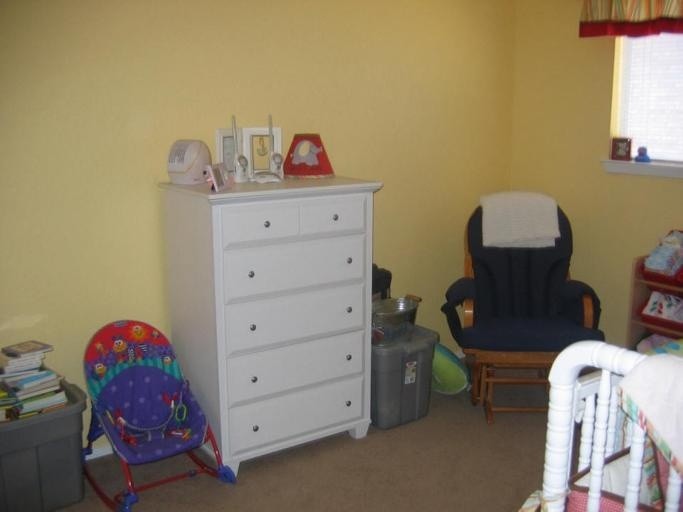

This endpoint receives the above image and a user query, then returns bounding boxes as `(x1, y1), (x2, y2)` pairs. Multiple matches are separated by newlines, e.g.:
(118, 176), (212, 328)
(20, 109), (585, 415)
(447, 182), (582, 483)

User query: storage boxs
(370, 325), (441, 430)
(0, 366), (88, 512)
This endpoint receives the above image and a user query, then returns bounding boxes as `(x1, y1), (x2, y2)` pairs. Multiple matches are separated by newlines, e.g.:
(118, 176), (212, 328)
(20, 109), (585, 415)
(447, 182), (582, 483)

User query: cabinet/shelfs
(625, 254), (683, 355)
(156, 178), (384, 478)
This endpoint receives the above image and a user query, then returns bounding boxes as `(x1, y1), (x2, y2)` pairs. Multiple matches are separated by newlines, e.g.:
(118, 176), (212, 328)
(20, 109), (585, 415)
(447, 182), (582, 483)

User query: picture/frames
(216, 127), (282, 181)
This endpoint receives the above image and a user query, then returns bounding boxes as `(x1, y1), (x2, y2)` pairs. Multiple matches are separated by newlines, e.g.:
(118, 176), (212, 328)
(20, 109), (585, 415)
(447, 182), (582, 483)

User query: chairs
(460, 195), (605, 424)
(80, 321), (237, 511)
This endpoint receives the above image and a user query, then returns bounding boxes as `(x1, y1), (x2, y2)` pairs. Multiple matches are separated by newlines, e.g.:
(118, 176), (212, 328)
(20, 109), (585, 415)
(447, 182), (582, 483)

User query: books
(0, 340), (68, 418)
